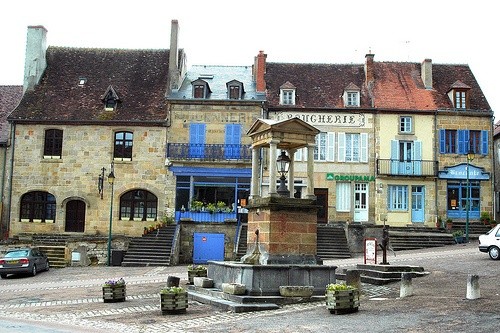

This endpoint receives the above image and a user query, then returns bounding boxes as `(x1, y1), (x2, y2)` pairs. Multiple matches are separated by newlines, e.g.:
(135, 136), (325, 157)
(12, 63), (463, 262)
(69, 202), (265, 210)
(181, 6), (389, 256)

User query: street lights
(107, 172), (116, 266)
(466, 150), (475, 241)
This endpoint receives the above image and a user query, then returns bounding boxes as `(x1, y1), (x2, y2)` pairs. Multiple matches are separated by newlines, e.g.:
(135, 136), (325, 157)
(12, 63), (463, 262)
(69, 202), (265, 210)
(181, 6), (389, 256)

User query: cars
(0, 247), (50, 279)
(478, 223), (500, 260)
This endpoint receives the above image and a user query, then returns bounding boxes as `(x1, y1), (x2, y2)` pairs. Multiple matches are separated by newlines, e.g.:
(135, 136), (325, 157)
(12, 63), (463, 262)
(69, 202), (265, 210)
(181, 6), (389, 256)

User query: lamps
(276, 151), (291, 196)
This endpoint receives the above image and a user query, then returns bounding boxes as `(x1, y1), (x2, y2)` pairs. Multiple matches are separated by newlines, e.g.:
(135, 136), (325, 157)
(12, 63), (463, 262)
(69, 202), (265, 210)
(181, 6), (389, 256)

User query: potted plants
(481, 212), (490, 225)
(436, 218), (442, 228)
(324, 283), (360, 315)
(491, 221), (497, 228)
(187, 264), (207, 284)
(143, 199), (236, 236)
(160, 286), (189, 314)
(446, 218), (453, 230)
(102, 278), (126, 303)
